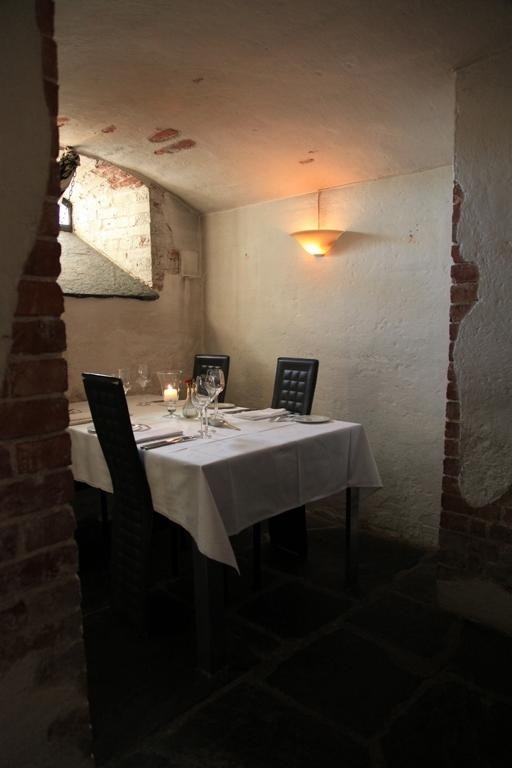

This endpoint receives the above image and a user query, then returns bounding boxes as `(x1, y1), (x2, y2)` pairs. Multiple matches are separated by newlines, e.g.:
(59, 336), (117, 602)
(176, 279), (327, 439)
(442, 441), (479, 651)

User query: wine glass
(190, 367), (226, 440)
(134, 363), (152, 406)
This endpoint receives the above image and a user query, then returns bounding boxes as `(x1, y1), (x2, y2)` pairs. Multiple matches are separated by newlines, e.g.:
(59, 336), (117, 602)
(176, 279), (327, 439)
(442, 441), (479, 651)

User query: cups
(156, 369), (183, 402)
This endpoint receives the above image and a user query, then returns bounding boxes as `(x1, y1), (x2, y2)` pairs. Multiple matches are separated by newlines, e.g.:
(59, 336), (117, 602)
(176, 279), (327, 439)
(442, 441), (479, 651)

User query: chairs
(191, 354), (230, 404)
(250, 357), (322, 562)
(81, 370), (221, 622)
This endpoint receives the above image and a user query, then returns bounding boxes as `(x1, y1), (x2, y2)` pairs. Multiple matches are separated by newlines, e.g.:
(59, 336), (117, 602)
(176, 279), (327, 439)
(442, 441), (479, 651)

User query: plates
(294, 413), (330, 424)
(207, 402), (235, 409)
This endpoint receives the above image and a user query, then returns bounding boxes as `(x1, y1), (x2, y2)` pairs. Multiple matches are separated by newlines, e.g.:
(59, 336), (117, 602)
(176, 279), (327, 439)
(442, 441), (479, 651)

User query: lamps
(290, 191), (345, 258)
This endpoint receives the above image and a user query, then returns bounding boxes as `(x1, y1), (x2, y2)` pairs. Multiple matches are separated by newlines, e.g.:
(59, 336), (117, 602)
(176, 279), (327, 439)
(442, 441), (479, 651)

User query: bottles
(182, 386), (197, 418)
